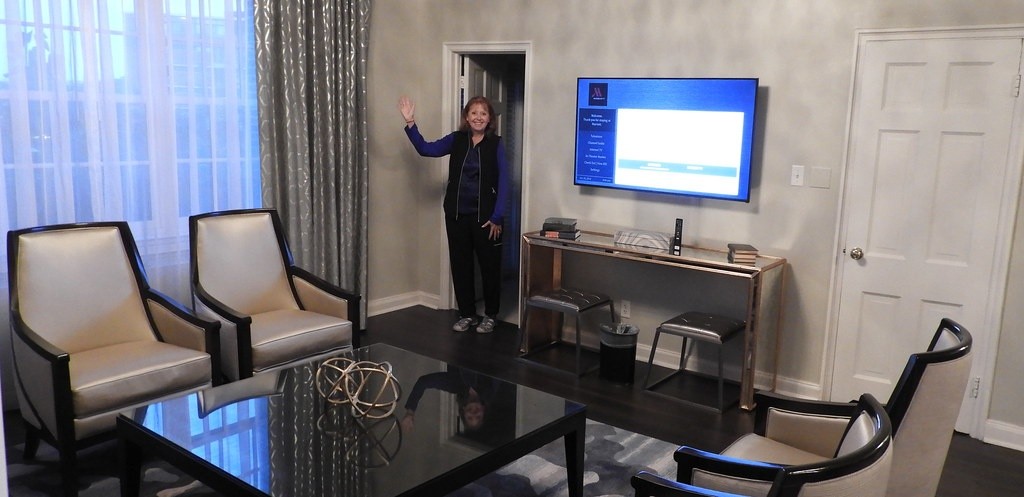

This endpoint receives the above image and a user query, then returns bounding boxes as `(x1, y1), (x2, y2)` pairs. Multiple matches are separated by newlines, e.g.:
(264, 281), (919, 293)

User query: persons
(400, 96), (510, 333)
(401, 362), (499, 432)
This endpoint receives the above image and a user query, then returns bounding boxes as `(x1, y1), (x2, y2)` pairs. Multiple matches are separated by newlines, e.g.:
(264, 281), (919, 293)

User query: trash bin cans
(600, 322), (639, 382)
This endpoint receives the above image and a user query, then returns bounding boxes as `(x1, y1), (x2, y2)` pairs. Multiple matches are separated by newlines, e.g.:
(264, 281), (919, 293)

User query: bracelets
(406, 120), (414, 123)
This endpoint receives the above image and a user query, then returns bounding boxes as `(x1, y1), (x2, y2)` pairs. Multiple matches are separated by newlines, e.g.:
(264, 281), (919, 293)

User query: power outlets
(620, 301), (631, 319)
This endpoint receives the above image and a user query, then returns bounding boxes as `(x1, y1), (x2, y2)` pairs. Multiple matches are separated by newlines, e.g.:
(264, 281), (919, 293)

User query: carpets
(7, 417), (700, 497)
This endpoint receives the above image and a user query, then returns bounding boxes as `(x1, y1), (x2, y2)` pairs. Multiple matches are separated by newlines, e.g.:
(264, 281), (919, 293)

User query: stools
(517, 287), (615, 378)
(640, 312), (750, 414)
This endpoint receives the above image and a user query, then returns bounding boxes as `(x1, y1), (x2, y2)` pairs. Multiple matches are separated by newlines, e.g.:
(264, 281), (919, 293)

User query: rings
(498, 229), (500, 231)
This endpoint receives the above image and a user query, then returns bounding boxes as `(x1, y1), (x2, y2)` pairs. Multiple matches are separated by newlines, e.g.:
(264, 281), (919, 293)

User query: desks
(522, 226), (787, 410)
(116, 344), (590, 497)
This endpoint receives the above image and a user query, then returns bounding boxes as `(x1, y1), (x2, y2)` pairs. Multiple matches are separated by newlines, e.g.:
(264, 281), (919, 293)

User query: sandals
(453, 314), (479, 331)
(476, 315), (496, 332)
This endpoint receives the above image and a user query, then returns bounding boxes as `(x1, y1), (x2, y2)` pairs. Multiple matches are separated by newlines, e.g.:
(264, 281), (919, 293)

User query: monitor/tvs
(574, 77), (759, 203)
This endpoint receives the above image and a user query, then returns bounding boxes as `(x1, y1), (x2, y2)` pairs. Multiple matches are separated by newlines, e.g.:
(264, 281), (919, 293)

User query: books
(727, 244), (759, 263)
(539, 217), (581, 240)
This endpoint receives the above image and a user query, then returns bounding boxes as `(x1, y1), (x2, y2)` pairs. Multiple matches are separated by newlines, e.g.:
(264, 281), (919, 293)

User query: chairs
(187, 210), (362, 381)
(851, 317), (974, 496)
(195, 368), (288, 419)
(631, 395), (896, 497)
(5, 220), (226, 497)
